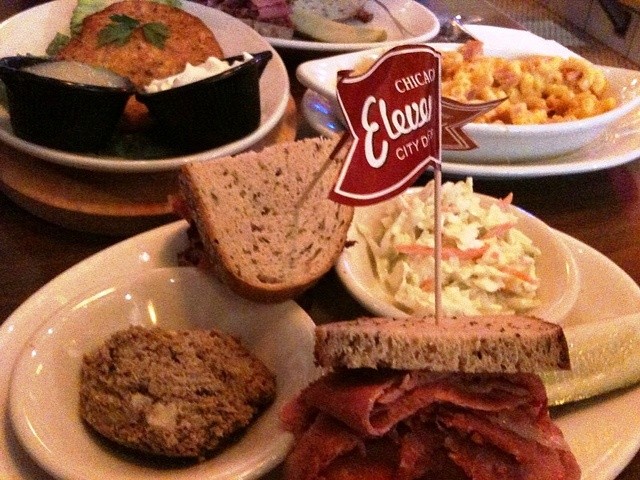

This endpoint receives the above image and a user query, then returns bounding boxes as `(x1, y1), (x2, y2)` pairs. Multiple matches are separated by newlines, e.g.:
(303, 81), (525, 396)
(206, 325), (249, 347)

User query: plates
(9, 265), (317, 478)
(2, 0), (289, 172)
(302, 22), (638, 179)
(4, 218), (312, 478)
(337, 184), (582, 328)
(297, 39), (639, 157)
(1, 187), (640, 479)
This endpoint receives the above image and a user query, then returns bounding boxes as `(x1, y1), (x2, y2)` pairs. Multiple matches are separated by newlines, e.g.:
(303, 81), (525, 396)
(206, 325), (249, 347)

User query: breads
(180, 137), (356, 303)
(79, 323), (279, 457)
(313, 314), (571, 373)
